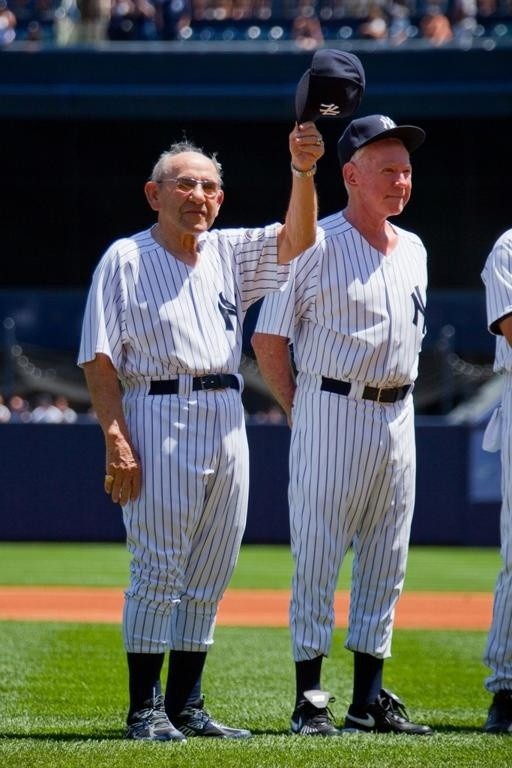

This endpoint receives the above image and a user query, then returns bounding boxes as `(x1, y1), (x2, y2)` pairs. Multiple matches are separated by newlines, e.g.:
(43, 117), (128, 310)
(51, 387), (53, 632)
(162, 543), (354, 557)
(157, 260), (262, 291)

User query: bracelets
(289, 161), (318, 178)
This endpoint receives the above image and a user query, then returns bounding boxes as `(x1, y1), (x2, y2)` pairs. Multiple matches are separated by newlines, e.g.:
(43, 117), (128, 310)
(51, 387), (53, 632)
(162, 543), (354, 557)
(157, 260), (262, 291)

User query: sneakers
(484, 689), (512, 732)
(343, 688), (433, 736)
(122, 686), (252, 743)
(289, 693), (342, 737)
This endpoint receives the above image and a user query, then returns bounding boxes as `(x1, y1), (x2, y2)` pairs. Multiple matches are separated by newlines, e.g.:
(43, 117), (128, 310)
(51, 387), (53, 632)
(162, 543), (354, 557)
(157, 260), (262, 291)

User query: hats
(294, 48), (366, 126)
(337, 114), (426, 168)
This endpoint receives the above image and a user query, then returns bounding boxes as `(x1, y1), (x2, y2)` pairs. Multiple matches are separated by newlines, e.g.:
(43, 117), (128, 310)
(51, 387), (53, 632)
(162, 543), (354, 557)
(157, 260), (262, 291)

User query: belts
(321, 376), (410, 402)
(149, 373), (239, 395)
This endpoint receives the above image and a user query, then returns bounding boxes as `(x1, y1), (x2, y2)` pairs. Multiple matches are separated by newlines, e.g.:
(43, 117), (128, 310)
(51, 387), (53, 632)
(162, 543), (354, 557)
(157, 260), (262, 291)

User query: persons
(73, 122), (327, 746)
(250, 113), (437, 743)
(55, 391), (77, 421)
(478, 220), (512, 742)
(31, 394), (63, 425)
(1, 3), (511, 52)
(0, 396), (12, 426)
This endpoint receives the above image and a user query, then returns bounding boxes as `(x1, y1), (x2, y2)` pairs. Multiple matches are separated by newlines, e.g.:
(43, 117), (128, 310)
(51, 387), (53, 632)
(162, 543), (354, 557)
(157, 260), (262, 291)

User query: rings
(316, 136), (323, 147)
(105, 474), (114, 483)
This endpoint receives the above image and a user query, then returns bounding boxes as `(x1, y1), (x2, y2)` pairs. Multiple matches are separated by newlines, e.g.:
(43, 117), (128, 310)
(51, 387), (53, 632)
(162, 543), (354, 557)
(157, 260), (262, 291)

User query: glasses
(156, 174), (219, 199)
